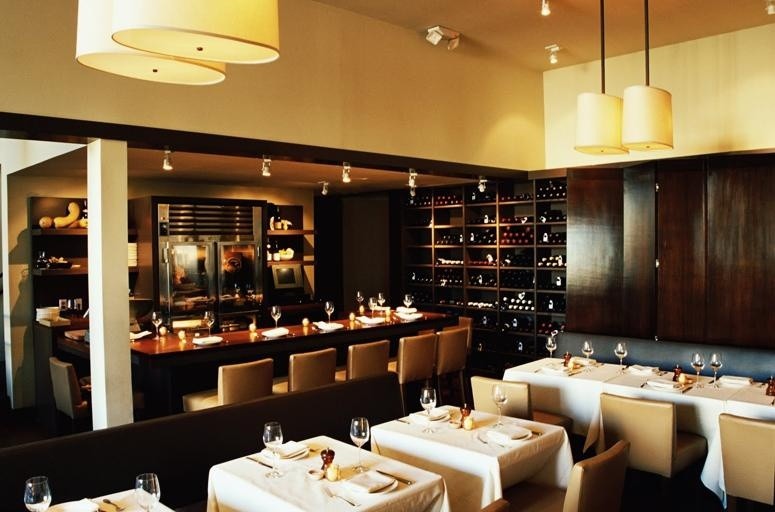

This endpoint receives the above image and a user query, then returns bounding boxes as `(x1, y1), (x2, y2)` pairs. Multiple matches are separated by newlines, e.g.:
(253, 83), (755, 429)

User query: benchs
(555, 332), (774, 384)
(0, 372), (403, 512)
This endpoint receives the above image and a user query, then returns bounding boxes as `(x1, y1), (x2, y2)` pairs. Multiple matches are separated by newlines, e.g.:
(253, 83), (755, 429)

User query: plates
(368, 479), (398, 494)
(192, 336), (223, 344)
(510, 430), (532, 442)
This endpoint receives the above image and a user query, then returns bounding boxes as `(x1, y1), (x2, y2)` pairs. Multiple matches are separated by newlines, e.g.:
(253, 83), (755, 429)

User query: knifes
(245, 456), (273, 469)
(377, 469), (412, 485)
(397, 419), (410, 425)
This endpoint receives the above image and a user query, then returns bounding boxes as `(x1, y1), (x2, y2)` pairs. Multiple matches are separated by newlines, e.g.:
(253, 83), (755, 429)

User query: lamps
(575, 0), (628, 155)
(75, 2), (227, 86)
(623, 1), (675, 152)
(111, 1), (280, 64)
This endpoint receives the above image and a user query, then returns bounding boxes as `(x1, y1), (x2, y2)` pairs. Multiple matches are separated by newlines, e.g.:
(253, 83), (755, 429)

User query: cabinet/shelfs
(401, 198), (567, 374)
(27, 197), (138, 435)
(267, 205), (318, 325)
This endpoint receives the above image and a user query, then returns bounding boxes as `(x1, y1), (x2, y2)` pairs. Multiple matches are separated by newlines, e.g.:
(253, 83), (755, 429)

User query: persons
(221, 257), (256, 295)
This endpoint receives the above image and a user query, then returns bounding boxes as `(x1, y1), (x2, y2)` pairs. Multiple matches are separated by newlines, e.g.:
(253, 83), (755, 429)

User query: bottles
(406, 176), (567, 351)
(273, 206), (281, 230)
(266, 237), (281, 262)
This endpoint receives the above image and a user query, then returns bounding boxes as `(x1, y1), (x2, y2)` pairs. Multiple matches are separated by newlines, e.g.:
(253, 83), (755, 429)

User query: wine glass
(23, 473), (161, 512)
(151, 283), (414, 340)
(546, 337), (628, 377)
(262, 417), (370, 479)
(420, 384), (508, 434)
(692, 352), (722, 389)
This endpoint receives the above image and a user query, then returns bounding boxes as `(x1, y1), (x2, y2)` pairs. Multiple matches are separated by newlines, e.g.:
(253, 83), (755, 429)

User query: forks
(321, 487), (356, 506)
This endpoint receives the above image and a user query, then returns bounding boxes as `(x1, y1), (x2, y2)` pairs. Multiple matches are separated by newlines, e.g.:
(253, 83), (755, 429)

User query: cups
(58, 299), (67, 310)
(74, 298), (82, 310)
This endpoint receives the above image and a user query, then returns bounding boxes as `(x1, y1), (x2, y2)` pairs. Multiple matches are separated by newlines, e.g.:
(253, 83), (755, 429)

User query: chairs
(180, 317), (474, 408)
(49, 356), (90, 432)
(470, 376), (572, 444)
